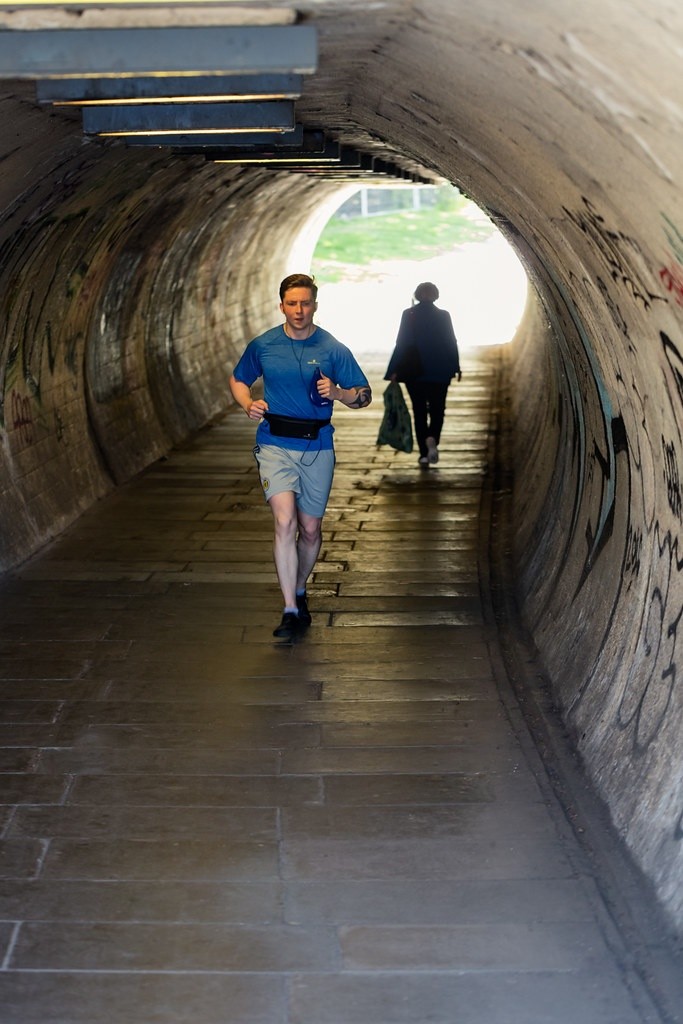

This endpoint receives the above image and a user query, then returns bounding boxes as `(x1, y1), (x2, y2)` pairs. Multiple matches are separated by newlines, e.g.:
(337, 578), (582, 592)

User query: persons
(229, 274), (371, 635)
(384, 281), (460, 464)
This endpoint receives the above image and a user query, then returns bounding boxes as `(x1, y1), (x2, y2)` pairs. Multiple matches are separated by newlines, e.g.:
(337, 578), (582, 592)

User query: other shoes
(426, 436), (439, 465)
(418, 455), (429, 467)
(273, 613), (302, 636)
(297, 591), (311, 630)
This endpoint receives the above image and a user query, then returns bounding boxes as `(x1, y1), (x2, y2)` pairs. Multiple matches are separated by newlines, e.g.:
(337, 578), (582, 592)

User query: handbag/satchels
(260, 413), (331, 440)
(376, 380), (413, 453)
